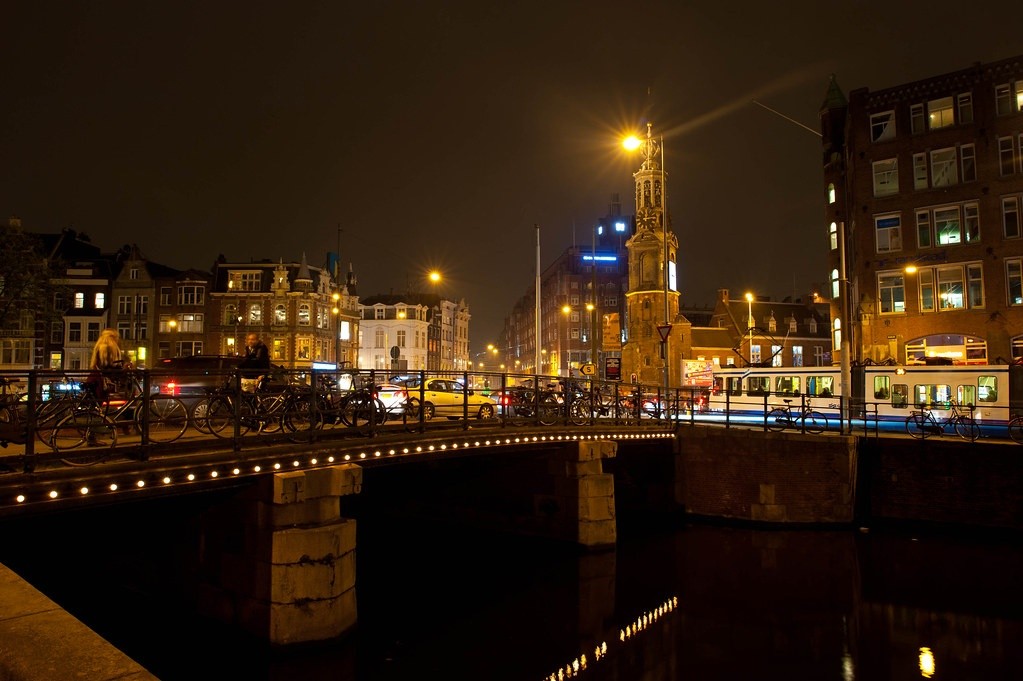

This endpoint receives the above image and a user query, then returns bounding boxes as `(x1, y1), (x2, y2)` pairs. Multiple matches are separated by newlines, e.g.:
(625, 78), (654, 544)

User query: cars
(489, 385), (559, 421)
(366, 371), (498, 422)
(142, 355), (310, 428)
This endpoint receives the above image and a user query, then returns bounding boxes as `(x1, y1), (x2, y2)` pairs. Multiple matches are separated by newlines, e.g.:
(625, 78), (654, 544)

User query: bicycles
(1007, 414), (1023, 444)
(0, 357), (422, 465)
(507, 378), (675, 427)
(904, 395), (982, 441)
(765, 394), (829, 436)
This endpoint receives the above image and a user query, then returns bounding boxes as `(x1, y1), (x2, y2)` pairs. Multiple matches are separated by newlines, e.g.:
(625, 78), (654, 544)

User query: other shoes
(87, 439), (107, 446)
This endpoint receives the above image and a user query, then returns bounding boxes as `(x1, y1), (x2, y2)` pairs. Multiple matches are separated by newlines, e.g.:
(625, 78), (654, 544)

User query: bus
(707, 326), (1011, 426)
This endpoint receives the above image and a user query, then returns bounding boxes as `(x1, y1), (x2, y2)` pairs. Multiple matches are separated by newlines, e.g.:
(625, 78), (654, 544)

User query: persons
(84, 329), (122, 446)
(819, 387), (831, 397)
(981, 387), (997, 401)
(241, 333), (270, 392)
(794, 390), (800, 397)
(875, 387), (906, 408)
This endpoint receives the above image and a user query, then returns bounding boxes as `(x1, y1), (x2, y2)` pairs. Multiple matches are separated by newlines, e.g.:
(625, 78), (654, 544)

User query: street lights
(624, 132), (674, 419)
(746, 291), (754, 364)
